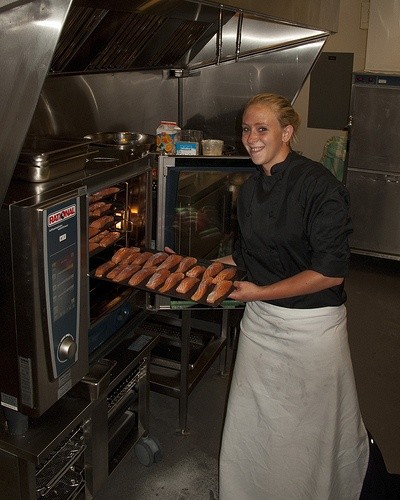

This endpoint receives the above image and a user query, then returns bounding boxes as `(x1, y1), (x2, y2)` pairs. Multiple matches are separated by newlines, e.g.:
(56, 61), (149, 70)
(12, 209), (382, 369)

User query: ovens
(0, 156), (261, 419)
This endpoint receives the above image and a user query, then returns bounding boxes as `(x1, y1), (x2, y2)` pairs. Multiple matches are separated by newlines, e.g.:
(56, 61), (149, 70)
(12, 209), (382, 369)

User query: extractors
(0, 0), (237, 204)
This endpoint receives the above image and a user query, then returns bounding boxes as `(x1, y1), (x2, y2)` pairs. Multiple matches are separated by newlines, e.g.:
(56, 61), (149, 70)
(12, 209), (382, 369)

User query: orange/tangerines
(157, 135), (173, 152)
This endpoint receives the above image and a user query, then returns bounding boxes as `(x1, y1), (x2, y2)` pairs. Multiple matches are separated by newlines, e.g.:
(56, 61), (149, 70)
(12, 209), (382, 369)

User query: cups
(179, 130), (203, 143)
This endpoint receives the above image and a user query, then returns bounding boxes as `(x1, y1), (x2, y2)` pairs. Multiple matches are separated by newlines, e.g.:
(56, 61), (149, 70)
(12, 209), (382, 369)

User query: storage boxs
(175, 141), (199, 157)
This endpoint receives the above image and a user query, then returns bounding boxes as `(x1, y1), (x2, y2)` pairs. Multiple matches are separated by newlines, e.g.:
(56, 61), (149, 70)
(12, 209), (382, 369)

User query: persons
(165, 93), (391, 500)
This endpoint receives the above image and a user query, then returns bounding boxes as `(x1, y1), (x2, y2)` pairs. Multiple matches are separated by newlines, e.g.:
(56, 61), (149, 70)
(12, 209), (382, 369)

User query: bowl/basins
(201, 139), (225, 156)
(84, 132), (157, 161)
